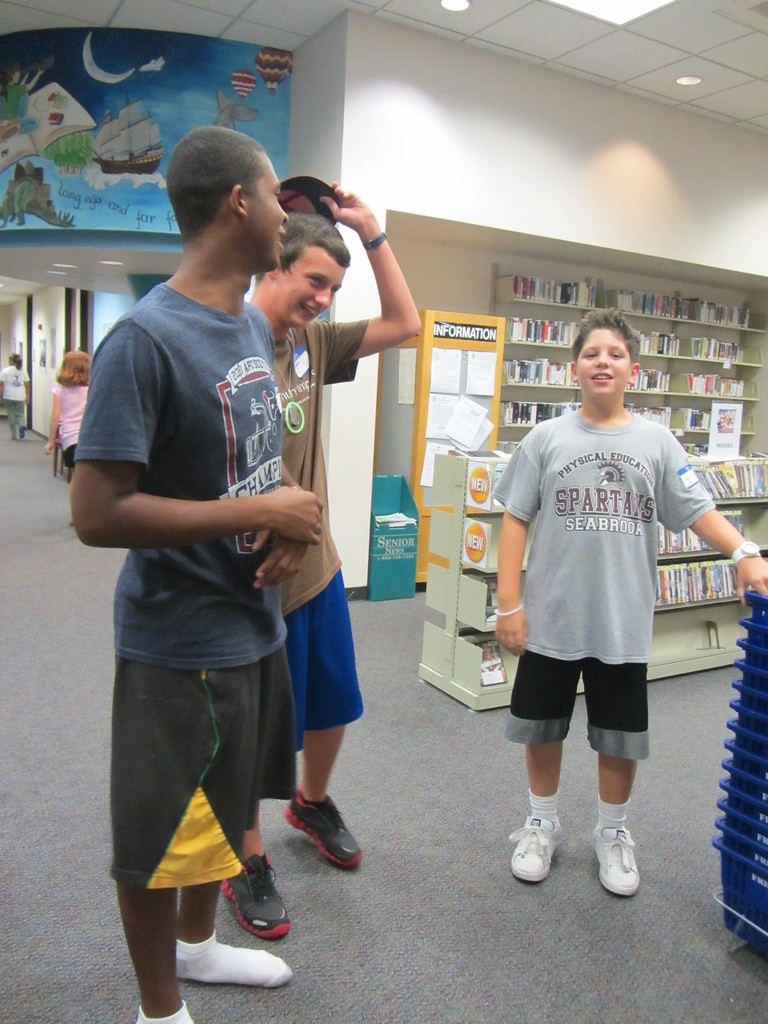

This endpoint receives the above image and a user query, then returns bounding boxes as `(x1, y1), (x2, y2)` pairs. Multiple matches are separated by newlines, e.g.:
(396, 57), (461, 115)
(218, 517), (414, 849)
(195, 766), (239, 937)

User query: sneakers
(222, 852), (291, 937)
(593, 824), (640, 896)
(508, 814), (563, 881)
(285, 785), (364, 868)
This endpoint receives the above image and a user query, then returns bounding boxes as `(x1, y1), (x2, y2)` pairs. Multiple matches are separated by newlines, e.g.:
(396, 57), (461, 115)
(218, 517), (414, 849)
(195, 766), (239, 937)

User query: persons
(45, 351), (92, 526)
(71, 127), (324, 1024)
(492, 309), (768, 896)
(221, 176), (421, 937)
(0, 354), (30, 439)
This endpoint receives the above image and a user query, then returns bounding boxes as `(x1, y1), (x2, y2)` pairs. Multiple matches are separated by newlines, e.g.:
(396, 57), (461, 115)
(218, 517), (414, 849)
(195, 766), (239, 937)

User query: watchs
(732, 542), (762, 565)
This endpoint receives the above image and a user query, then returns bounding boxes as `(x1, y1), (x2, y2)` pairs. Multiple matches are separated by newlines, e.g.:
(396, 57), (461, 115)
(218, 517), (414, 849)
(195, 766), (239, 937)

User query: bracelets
(363, 232), (386, 249)
(494, 604), (523, 616)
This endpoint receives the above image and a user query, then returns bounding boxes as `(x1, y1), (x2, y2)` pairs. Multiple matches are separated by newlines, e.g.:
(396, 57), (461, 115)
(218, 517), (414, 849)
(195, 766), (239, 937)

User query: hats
(277, 176), (342, 226)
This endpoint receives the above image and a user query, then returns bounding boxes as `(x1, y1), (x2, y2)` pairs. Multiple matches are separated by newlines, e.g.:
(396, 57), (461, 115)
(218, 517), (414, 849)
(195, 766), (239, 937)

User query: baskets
(710, 590), (768, 958)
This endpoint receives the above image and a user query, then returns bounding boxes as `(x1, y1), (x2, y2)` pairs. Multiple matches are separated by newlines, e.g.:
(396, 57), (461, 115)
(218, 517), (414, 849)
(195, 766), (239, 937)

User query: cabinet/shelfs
(420, 456), (768, 714)
(490, 267), (768, 455)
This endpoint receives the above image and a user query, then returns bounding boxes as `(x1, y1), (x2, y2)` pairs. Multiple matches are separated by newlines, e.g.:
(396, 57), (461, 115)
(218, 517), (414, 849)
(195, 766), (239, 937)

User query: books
(688, 372), (744, 397)
(514, 275), (750, 328)
(692, 336), (738, 361)
(509, 317), (579, 345)
(484, 577), (499, 622)
(501, 400), (583, 424)
(656, 510), (744, 555)
(636, 330), (680, 355)
(689, 460), (767, 498)
(627, 369), (670, 391)
(655, 559), (753, 606)
(625, 405), (671, 427)
(476, 641), (507, 687)
(503, 358), (579, 387)
(679, 406), (768, 458)
(448, 441), (521, 507)
